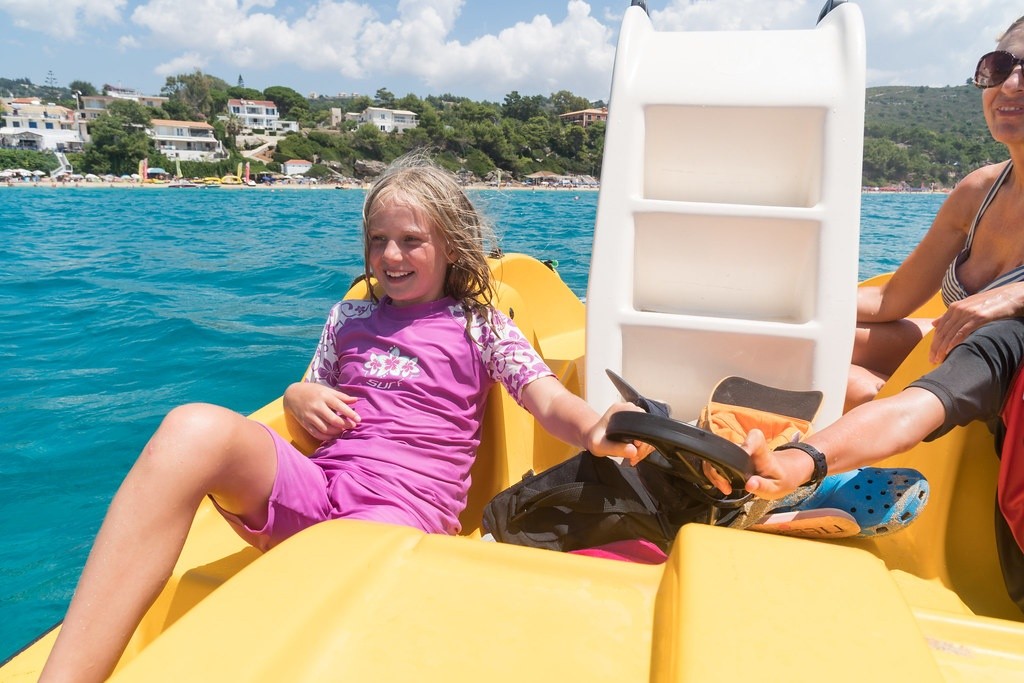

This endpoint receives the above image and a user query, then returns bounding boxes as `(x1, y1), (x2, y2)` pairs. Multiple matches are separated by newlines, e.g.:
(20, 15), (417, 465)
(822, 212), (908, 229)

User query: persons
(38, 149), (657, 683)
(702, 17), (1024, 617)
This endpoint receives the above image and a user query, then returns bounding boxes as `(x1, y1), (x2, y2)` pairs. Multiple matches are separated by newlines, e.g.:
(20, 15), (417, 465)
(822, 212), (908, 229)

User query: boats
(167, 176), (256, 188)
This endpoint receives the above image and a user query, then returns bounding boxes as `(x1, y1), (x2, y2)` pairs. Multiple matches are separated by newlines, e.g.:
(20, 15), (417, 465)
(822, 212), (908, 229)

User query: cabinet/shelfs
(584, 0), (866, 433)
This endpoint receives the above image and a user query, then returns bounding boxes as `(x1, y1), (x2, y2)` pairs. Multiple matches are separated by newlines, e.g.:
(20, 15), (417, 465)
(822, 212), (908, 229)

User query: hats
(699, 375), (824, 483)
(606, 368), (670, 417)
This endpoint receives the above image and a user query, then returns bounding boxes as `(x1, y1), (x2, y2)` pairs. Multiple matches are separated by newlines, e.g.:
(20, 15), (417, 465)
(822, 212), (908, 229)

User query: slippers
(770, 467), (933, 538)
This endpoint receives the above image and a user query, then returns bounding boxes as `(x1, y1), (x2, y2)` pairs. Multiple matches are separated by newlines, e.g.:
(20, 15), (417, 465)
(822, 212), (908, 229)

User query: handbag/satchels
(483, 451), (715, 553)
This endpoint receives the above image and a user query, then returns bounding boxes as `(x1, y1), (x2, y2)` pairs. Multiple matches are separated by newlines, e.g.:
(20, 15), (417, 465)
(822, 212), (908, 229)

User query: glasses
(971, 51), (1024, 89)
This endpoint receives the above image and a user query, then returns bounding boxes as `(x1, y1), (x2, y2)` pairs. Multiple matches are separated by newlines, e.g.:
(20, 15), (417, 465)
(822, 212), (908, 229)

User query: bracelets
(772, 442), (828, 487)
